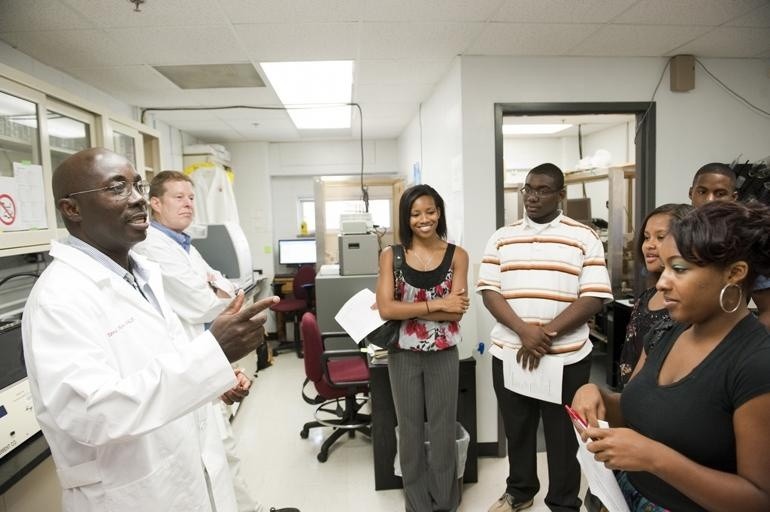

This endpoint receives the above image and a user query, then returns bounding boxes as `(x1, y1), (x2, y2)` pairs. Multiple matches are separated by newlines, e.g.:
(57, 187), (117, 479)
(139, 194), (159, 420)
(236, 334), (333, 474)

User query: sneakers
(488, 492), (534, 512)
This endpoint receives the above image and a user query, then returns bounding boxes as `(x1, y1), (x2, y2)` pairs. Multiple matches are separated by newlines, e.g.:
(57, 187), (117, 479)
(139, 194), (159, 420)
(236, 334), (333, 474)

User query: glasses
(63, 180), (151, 197)
(520, 187), (563, 198)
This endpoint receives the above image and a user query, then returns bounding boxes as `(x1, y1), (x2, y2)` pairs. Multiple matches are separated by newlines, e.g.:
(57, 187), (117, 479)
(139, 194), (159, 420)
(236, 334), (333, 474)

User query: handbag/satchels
(365, 278), (402, 352)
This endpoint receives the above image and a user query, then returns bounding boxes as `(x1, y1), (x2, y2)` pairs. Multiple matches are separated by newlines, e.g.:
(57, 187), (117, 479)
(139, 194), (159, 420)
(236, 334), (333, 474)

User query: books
(369, 341), (389, 367)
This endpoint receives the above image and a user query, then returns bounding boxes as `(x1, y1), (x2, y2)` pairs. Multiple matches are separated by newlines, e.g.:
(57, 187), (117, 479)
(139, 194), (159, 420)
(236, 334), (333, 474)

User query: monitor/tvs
(278, 239), (316, 265)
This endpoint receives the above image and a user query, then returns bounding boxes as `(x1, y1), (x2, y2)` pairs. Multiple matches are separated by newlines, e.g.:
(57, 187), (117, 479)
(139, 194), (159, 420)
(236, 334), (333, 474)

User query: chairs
(301, 312), (371, 463)
(270, 266), (316, 358)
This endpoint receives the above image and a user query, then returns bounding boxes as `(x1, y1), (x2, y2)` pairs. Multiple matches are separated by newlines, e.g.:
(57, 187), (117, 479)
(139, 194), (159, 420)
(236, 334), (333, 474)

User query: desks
(272, 278), (293, 343)
(366, 349), (477, 491)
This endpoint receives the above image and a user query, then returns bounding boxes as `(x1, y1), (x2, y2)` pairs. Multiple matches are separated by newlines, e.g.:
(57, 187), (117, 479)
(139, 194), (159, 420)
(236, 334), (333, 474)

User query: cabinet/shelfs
(314, 264), (377, 360)
(504, 164), (636, 390)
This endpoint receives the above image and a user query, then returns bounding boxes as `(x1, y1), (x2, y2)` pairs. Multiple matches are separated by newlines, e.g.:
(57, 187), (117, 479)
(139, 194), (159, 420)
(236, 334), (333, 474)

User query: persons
(370, 185), (469, 511)
(689, 163), (769, 326)
(20, 147), (281, 512)
(126, 171), (261, 421)
(478, 163), (604, 512)
(571, 202), (769, 512)
(615, 203), (694, 393)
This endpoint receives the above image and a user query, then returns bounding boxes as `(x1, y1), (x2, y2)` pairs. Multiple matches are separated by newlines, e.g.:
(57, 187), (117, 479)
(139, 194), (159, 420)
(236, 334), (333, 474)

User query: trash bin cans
(394, 421), (470, 507)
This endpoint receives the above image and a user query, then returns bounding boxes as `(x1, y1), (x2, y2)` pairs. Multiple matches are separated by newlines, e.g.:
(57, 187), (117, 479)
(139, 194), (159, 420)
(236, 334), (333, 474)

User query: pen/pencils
(565, 405), (598, 442)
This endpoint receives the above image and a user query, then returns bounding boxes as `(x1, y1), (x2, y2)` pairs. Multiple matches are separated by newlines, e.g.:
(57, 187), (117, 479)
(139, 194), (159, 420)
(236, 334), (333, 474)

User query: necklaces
(408, 240), (440, 272)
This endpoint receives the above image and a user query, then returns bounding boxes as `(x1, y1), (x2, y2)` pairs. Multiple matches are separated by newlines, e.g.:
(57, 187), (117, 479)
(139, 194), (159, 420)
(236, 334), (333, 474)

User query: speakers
(670, 54), (695, 93)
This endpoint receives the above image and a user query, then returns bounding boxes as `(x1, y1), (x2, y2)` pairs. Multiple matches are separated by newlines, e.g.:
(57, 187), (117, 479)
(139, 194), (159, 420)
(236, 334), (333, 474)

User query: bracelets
(425, 300), (430, 315)
